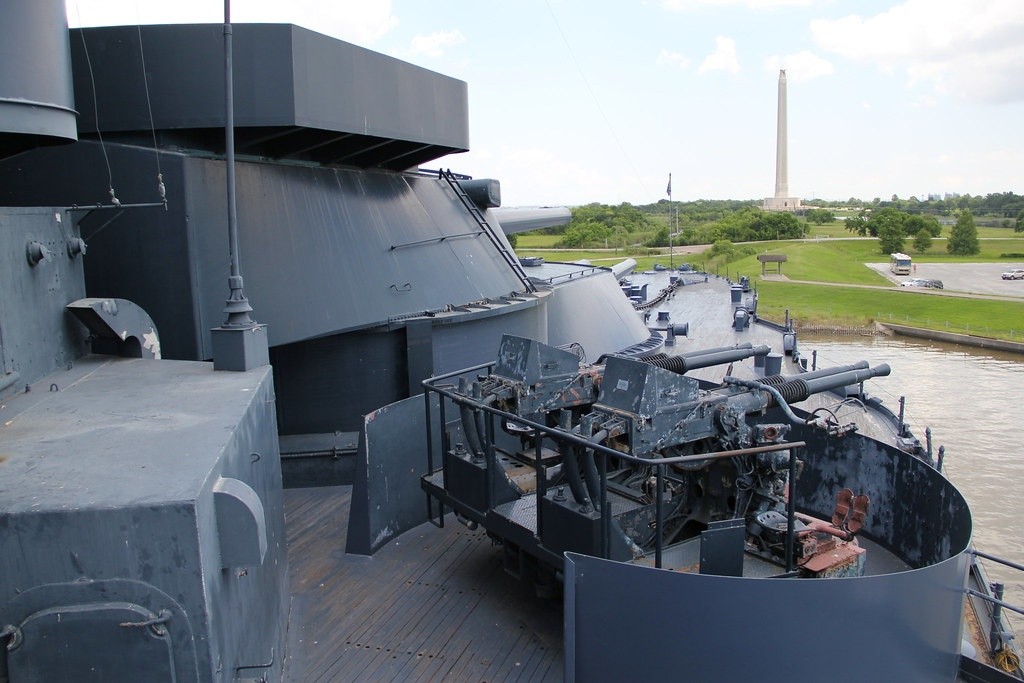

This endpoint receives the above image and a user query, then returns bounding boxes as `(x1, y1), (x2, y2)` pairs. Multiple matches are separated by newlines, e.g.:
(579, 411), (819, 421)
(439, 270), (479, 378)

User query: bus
(890, 253), (911, 275)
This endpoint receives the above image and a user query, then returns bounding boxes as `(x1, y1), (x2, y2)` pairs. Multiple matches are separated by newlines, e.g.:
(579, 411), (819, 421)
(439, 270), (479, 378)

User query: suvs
(1002, 269), (1024, 280)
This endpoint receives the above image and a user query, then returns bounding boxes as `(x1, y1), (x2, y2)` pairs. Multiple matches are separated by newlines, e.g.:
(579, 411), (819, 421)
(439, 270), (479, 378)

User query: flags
(666, 174), (671, 195)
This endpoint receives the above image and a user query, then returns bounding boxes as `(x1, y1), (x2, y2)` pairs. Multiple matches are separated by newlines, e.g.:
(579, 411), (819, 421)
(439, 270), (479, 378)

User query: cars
(901, 278), (943, 290)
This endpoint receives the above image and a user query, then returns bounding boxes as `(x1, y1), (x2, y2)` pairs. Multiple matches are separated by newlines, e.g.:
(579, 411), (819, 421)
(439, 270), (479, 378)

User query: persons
(914, 264), (917, 273)
